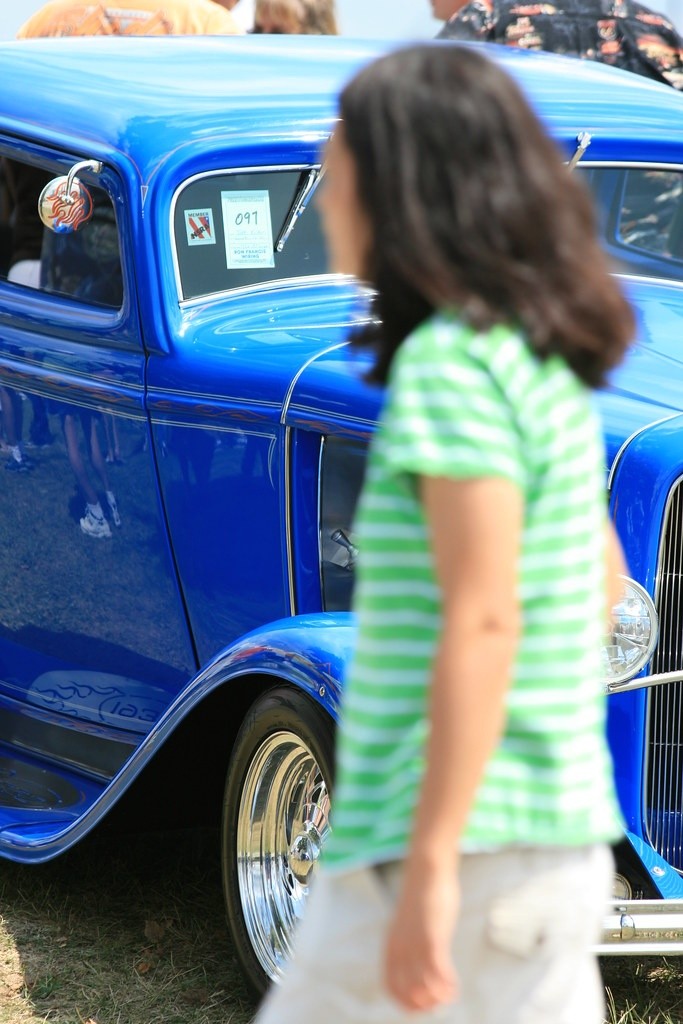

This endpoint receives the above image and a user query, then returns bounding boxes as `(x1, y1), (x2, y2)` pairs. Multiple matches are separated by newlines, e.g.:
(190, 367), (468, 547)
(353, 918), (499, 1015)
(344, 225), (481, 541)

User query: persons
(253, 38), (643, 1024)
(17, 0), (683, 258)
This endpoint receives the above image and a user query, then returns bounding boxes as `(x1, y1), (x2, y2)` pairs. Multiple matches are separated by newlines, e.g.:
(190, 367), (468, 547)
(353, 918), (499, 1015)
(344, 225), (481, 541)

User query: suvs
(0, 35), (683, 996)
